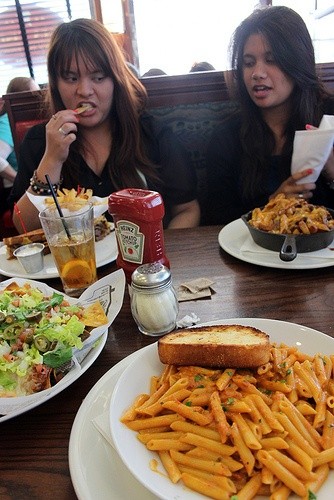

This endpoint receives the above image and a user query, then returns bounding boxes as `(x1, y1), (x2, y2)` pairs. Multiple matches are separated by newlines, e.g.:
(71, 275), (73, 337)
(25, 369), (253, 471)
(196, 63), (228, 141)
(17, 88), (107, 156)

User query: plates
(67, 317), (334, 500)
(0, 221), (120, 280)
(0, 298), (109, 424)
(112, 345), (334, 500)
(218, 217), (334, 269)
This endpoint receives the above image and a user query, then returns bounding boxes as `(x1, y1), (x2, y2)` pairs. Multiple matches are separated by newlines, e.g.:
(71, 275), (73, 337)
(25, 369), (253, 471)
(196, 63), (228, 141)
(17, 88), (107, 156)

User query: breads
(158, 325), (271, 367)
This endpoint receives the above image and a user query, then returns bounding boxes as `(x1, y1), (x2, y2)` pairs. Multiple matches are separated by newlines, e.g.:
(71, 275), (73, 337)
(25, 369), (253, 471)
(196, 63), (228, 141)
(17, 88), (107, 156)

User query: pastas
(251, 193), (334, 234)
(120, 342), (334, 500)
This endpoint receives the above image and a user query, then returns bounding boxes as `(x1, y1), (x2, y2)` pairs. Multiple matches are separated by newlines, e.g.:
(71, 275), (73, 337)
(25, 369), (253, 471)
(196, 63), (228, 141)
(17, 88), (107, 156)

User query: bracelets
(30, 170), (63, 198)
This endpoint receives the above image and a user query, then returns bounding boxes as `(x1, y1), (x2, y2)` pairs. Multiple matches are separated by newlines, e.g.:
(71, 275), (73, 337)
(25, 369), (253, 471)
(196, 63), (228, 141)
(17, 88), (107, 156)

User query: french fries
(75, 106), (90, 113)
(45, 188), (94, 212)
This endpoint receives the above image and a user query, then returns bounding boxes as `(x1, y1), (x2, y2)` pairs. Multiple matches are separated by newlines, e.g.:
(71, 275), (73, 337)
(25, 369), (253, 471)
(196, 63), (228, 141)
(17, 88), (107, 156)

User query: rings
(51, 114), (56, 120)
(58, 127), (67, 136)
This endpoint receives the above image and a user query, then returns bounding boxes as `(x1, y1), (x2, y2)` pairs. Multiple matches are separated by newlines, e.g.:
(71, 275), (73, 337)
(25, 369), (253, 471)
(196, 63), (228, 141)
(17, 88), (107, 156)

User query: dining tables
(1, 223), (334, 500)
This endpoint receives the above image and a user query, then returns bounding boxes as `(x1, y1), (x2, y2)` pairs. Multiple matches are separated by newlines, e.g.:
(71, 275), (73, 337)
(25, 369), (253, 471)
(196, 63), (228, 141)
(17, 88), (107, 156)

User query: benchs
(2, 60), (334, 229)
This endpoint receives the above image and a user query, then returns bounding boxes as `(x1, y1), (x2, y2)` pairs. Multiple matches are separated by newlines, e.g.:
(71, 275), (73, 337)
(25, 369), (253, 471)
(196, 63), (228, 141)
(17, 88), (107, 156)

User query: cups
(38, 201), (98, 298)
(13, 242), (45, 275)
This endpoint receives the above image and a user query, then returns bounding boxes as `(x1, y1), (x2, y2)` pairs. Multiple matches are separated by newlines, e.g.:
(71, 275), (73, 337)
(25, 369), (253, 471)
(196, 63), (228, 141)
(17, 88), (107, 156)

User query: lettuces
(0, 285), (86, 394)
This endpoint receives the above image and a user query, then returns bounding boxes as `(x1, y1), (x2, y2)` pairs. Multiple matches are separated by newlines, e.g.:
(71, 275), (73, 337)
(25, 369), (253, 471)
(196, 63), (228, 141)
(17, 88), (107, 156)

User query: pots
(241, 205), (334, 262)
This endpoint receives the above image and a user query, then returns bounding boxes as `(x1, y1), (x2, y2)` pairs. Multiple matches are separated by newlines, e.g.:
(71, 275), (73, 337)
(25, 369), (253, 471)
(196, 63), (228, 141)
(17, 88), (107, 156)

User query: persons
(201, 6), (334, 227)
(0, 77), (41, 208)
(125, 61), (215, 78)
(13, 19), (200, 235)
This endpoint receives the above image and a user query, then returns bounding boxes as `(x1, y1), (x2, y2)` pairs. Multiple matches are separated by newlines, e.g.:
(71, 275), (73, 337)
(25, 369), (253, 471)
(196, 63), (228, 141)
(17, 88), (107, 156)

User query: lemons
(87, 259), (96, 280)
(62, 261), (92, 287)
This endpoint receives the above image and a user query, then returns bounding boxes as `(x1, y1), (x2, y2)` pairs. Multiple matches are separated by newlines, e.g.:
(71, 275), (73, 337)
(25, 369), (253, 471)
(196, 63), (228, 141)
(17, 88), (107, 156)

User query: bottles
(108, 187), (171, 297)
(130, 264), (179, 336)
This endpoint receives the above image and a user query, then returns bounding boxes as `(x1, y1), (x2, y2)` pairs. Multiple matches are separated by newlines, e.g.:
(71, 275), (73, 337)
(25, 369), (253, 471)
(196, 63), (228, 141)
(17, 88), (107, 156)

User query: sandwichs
(4, 227), (51, 259)
